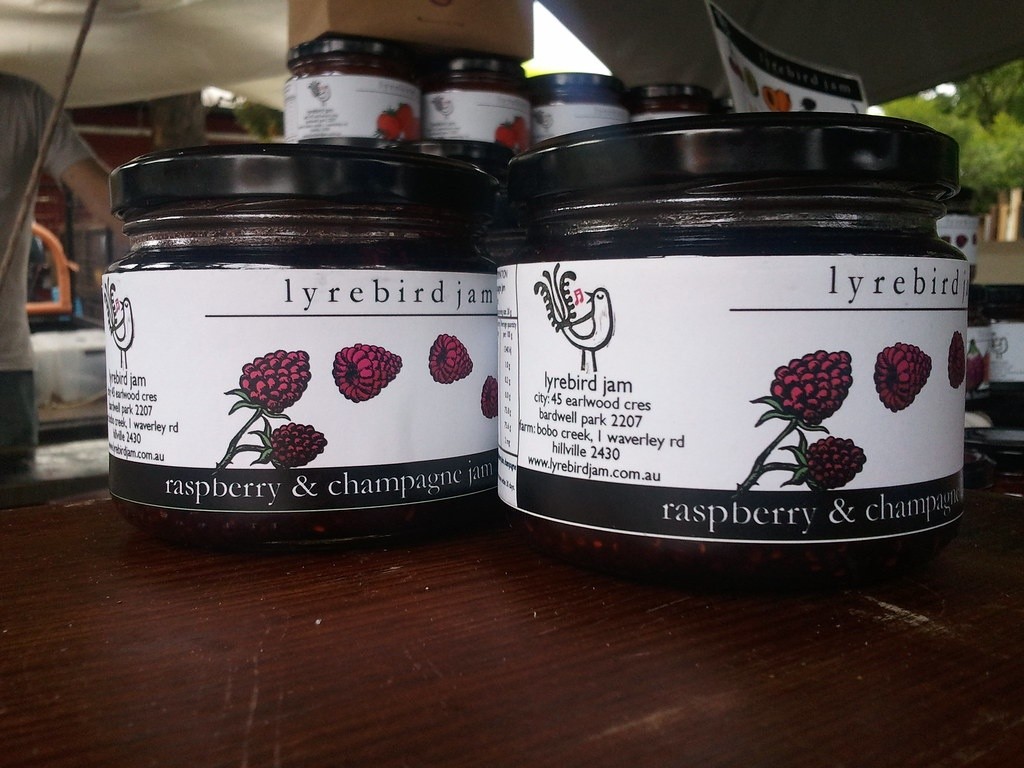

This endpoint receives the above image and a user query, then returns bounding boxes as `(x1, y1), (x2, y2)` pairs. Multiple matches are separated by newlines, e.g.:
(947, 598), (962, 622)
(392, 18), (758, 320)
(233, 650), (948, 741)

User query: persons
(0, 70), (129, 510)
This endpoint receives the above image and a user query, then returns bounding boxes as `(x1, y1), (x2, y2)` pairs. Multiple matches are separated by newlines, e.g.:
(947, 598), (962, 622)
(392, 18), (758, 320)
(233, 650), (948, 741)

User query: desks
(0, 487), (1024, 768)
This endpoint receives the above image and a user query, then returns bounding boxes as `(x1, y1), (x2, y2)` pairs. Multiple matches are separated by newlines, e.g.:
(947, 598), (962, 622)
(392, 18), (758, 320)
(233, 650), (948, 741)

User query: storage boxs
(287, 0), (534, 62)
(30, 328), (107, 405)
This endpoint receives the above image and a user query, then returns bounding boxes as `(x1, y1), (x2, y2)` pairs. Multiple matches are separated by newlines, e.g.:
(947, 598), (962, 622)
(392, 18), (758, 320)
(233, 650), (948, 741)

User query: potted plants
(879, 59), (1024, 214)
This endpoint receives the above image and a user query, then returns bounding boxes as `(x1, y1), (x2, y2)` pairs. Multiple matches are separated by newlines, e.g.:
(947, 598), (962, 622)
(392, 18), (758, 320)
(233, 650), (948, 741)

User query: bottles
(98, 30), (1024, 589)
